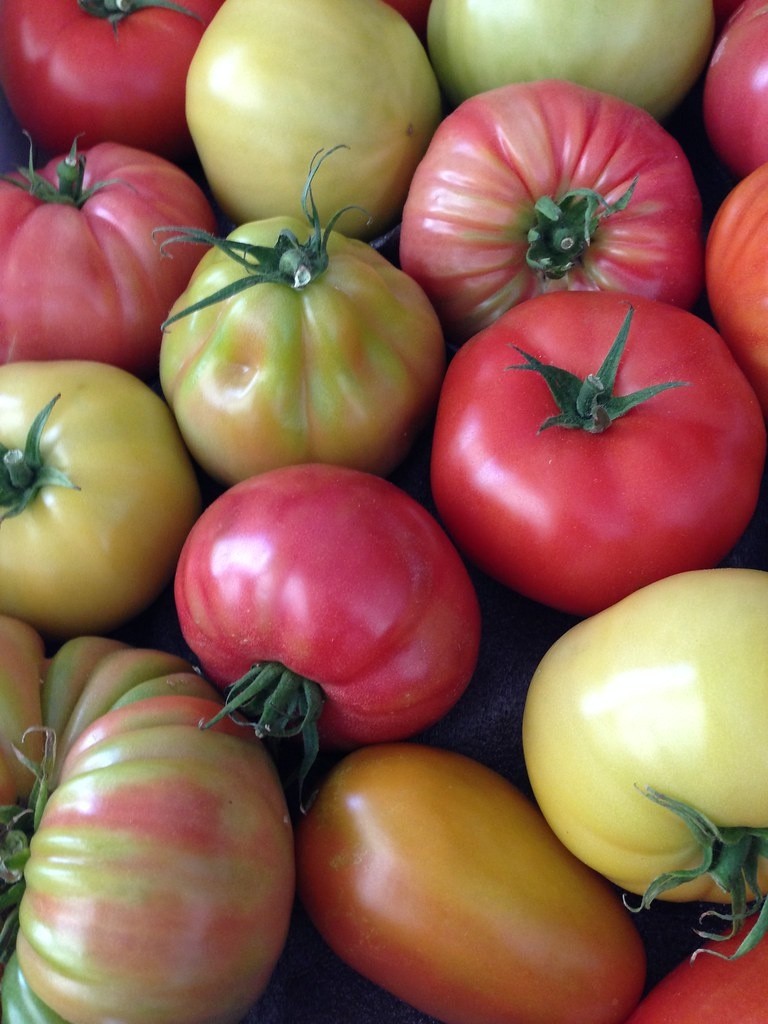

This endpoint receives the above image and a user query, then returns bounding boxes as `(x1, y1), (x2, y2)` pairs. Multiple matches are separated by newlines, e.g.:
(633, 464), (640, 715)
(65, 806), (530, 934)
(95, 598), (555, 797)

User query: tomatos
(0, 0), (768, 1024)
